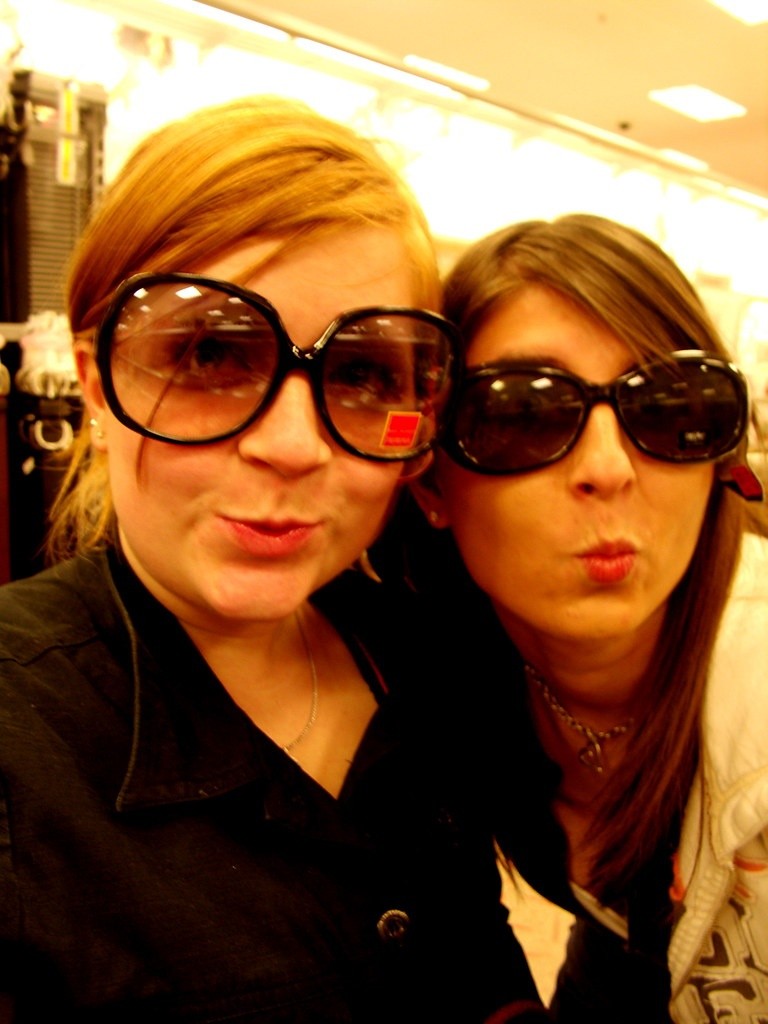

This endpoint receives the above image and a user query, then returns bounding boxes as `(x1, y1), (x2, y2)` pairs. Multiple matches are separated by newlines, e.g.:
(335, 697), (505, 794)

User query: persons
(0, 96), (768, 1024)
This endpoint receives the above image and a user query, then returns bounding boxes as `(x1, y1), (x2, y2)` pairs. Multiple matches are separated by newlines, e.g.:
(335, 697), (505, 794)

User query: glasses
(435, 349), (749, 476)
(73, 271), (452, 463)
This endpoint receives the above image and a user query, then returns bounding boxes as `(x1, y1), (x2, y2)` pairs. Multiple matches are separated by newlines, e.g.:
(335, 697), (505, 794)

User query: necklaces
(529, 665), (633, 775)
(278, 610), (321, 747)
